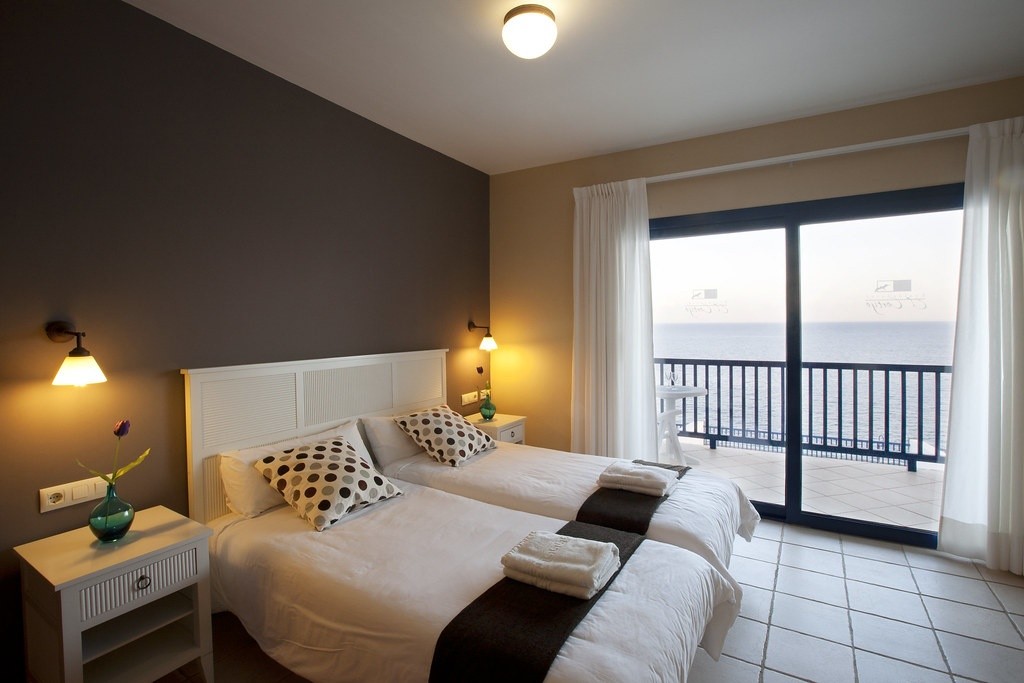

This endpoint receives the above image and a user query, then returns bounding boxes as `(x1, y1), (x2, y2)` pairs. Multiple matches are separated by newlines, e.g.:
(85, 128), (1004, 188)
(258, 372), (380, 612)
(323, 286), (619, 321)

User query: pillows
(393, 403), (498, 467)
(361, 417), (426, 469)
(216, 418), (375, 520)
(254, 435), (403, 532)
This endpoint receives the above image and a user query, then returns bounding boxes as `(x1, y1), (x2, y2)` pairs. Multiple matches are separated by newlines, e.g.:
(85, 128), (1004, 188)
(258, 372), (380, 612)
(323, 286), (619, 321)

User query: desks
(653, 385), (708, 467)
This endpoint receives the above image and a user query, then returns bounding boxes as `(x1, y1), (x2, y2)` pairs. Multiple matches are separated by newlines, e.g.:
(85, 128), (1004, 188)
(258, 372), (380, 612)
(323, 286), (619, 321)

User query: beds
(177, 347), (764, 683)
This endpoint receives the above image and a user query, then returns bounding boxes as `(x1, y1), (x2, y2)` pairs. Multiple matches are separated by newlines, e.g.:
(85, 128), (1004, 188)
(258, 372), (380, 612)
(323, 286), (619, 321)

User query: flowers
(75, 419), (152, 529)
(474, 365), (491, 417)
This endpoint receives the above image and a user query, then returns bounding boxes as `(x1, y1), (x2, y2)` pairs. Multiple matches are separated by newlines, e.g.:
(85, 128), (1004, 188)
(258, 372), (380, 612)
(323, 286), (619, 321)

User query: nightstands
(11, 503), (215, 683)
(463, 411), (528, 445)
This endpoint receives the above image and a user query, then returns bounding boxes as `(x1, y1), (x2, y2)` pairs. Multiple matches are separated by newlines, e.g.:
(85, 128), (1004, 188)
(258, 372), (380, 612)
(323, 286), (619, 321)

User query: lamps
(502, 4), (558, 60)
(44, 320), (109, 387)
(468, 320), (499, 352)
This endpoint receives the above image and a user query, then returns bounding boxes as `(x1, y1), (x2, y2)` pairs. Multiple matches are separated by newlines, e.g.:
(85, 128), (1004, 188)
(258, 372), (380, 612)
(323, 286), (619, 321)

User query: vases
(88, 484), (136, 542)
(479, 395), (496, 420)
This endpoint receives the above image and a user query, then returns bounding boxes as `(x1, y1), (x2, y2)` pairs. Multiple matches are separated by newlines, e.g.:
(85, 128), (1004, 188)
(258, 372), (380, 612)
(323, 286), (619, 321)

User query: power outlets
(39, 485), (70, 514)
(473, 391), (478, 403)
